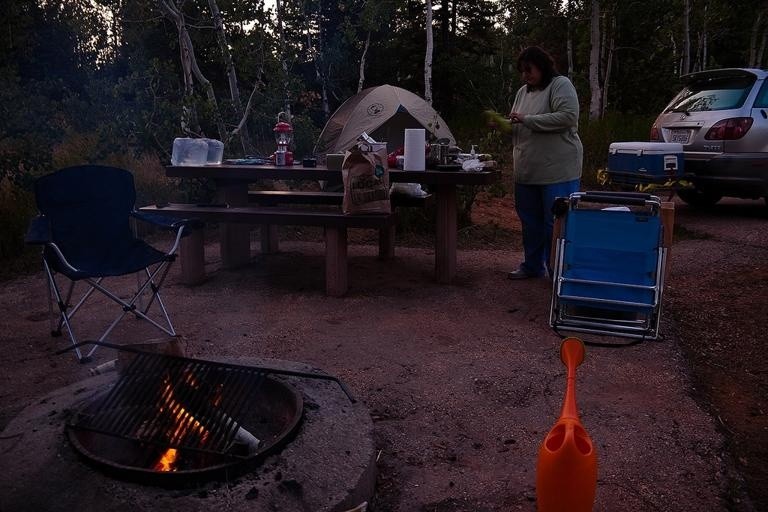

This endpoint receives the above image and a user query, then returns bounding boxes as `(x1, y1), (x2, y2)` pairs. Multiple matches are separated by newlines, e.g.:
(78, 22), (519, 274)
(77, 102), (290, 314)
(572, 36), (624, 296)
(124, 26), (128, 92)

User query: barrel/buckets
(170, 136), (224, 167)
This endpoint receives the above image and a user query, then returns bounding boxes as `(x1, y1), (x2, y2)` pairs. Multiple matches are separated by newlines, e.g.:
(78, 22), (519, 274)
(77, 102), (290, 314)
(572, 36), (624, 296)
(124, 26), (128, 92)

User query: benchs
(137, 198), (391, 297)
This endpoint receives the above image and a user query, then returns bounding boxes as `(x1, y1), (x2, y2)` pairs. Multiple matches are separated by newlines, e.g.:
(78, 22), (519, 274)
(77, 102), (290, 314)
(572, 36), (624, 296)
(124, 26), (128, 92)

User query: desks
(166, 161), (496, 298)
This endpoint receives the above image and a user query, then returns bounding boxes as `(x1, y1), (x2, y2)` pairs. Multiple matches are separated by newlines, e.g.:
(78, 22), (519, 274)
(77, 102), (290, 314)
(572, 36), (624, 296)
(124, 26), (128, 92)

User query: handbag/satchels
(341, 141), (393, 216)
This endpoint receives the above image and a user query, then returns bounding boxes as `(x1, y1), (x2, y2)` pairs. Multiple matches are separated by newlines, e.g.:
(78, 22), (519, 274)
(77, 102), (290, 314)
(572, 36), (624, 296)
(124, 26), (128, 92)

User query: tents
(311, 84), (458, 165)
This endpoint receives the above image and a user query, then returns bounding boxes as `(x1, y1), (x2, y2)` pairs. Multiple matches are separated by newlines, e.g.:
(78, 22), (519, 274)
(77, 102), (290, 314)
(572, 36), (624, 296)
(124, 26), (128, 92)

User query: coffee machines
(273, 122), (293, 166)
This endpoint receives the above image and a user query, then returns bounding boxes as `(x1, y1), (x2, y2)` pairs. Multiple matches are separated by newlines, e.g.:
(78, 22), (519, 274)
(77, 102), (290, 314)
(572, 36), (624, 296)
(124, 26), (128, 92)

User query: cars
(649, 67), (767, 206)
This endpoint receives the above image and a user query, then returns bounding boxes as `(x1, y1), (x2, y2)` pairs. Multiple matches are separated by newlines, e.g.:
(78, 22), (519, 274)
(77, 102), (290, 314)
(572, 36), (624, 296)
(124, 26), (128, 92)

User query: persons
(487, 46), (584, 280)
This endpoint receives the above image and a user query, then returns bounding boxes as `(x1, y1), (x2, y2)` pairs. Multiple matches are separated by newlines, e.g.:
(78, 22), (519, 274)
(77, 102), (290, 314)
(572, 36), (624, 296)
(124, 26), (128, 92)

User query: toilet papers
(404, 129), (426, 171)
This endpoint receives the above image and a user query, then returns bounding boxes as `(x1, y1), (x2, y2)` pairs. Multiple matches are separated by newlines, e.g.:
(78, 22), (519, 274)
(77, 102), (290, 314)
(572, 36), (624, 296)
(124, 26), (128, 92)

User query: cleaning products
(469, 145), (478, 160)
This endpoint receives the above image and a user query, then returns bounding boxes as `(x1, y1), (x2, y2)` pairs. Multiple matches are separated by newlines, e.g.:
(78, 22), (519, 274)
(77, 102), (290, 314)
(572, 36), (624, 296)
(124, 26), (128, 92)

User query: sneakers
(508, 264), (545, 282)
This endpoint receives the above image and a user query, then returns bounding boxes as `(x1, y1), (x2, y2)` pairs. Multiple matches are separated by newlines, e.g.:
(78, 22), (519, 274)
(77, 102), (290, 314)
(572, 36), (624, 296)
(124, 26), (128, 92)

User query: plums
(425, 153), (430, 161)
(424, 144), (431, 152)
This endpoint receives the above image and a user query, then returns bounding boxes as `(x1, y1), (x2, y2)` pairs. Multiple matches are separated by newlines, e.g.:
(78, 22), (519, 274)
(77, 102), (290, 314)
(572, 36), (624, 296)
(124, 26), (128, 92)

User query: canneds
(430, 144), (440, 165)
(439, 145), (450, 166)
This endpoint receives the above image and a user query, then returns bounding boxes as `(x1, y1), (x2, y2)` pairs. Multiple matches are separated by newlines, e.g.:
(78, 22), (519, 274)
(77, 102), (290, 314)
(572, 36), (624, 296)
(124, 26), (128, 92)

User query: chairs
(551, 191), (675, 349)
(30, 165), (191, 364)
